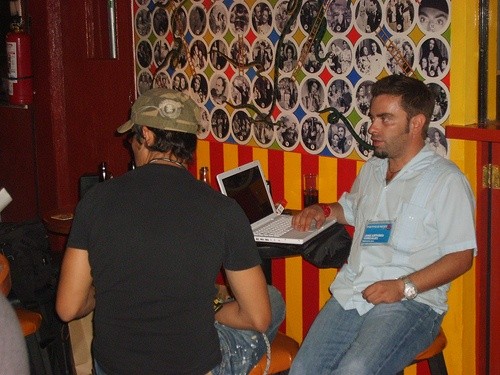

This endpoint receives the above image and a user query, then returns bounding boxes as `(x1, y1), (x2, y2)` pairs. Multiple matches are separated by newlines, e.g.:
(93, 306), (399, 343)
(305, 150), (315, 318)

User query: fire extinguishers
(5, 14), (33, 106)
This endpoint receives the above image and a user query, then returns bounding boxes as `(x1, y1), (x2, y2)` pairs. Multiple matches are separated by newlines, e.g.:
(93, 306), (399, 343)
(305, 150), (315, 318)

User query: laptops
(216, 160), (338, 244)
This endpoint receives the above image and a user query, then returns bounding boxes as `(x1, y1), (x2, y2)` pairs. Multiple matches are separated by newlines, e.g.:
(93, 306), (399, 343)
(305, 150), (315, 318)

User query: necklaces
(146, 158), (183, 167)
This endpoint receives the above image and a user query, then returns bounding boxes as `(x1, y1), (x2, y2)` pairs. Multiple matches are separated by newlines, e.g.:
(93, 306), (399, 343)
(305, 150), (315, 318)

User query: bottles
(200, 166), (209, 185)
(130, 162), (136, 172)
(304, 173), (319, 209)
(99, 161), (109, 182)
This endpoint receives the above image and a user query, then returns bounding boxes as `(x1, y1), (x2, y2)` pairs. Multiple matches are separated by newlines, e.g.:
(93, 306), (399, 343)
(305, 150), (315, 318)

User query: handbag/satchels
(0, 218), (51, 297)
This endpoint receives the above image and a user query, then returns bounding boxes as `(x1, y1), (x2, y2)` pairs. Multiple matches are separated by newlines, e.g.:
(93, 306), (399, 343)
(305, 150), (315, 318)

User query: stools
(395, 328), (449, 375)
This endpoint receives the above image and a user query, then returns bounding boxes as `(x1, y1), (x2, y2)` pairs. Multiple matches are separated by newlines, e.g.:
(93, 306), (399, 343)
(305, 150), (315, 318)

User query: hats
(117, 88), (197, 136)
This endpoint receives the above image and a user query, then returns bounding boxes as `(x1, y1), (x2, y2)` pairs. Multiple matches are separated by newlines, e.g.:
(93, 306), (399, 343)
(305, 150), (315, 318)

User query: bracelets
(316, 202), (331, 218)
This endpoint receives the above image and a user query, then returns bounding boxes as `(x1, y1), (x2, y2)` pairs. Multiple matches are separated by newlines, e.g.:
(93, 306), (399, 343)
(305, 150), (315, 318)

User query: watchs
(398, 275), (418, 300)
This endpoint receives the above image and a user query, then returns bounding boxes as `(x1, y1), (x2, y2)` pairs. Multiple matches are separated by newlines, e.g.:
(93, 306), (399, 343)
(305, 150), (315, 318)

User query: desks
(41, 207), (342, 287)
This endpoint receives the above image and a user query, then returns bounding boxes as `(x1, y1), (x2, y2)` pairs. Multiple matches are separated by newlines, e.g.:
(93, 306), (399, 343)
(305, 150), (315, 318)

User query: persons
(55, 87), (285, 375)
(135, 0), (448, 160)
(288, 74), (478, 375)
